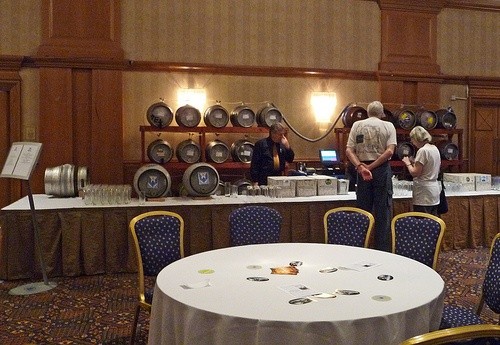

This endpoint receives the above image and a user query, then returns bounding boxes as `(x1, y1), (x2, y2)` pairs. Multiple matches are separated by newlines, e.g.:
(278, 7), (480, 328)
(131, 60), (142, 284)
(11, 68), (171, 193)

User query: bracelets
(406, 163), (412, 168)
(355, 165), (360, 171)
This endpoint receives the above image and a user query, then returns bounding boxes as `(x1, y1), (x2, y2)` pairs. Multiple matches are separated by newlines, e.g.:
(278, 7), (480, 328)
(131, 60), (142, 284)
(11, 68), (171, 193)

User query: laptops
(319, 150), (343, 165)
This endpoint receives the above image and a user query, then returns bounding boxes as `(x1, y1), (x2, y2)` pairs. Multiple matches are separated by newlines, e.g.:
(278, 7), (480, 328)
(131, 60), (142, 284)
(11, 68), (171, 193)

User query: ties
(273, 143), (279, 169)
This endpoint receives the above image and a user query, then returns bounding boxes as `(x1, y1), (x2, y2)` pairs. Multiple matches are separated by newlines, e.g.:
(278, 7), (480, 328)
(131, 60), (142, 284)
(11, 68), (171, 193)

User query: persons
(402, 126), (441, 218)
(344, 101), (397, 253)
(249, 123), (295, 186)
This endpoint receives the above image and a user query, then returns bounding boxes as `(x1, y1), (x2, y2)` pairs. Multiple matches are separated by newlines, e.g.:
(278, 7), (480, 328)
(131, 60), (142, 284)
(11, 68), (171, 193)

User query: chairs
(129, 207), (500, 345)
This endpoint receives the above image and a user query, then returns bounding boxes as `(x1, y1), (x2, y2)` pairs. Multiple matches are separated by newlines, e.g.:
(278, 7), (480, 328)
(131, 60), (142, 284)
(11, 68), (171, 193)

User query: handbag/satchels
(438, 187), (449, 215)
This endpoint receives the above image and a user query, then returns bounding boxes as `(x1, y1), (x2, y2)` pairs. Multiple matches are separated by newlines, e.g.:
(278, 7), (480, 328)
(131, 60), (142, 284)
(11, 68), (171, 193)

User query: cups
(392, 180), (413, 197)
(479, 176), (500, 193)
(242, 185), (283, 200)
(444, 181), (466, 194)
(138, 191), (145, 204)
(225, 182), (237, 198)
(83, 183), (132, 205)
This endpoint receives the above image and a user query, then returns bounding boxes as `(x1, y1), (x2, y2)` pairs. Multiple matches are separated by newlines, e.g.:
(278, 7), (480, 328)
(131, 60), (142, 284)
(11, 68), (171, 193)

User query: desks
(148, 243), (445, 345)
(0, 182), (500, 282)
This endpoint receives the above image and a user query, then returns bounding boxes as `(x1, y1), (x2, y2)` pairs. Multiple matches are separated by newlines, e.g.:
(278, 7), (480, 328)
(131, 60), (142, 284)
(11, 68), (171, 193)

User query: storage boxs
(443, 173), (491, 192)
(267, 174), (350, 198)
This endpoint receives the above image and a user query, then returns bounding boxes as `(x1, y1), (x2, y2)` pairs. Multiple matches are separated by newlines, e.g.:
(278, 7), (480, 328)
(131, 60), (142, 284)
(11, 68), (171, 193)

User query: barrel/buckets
(394, 141), (415, 160)
(147, 139), (256, 163)
(234, 179), (254, 194)
(133, 163), (228, 197)
(342, 106), (457, 130)
(44, 163), (88, 196)
(146, 101), (283, 127)
(433, 140), (458, 160)
